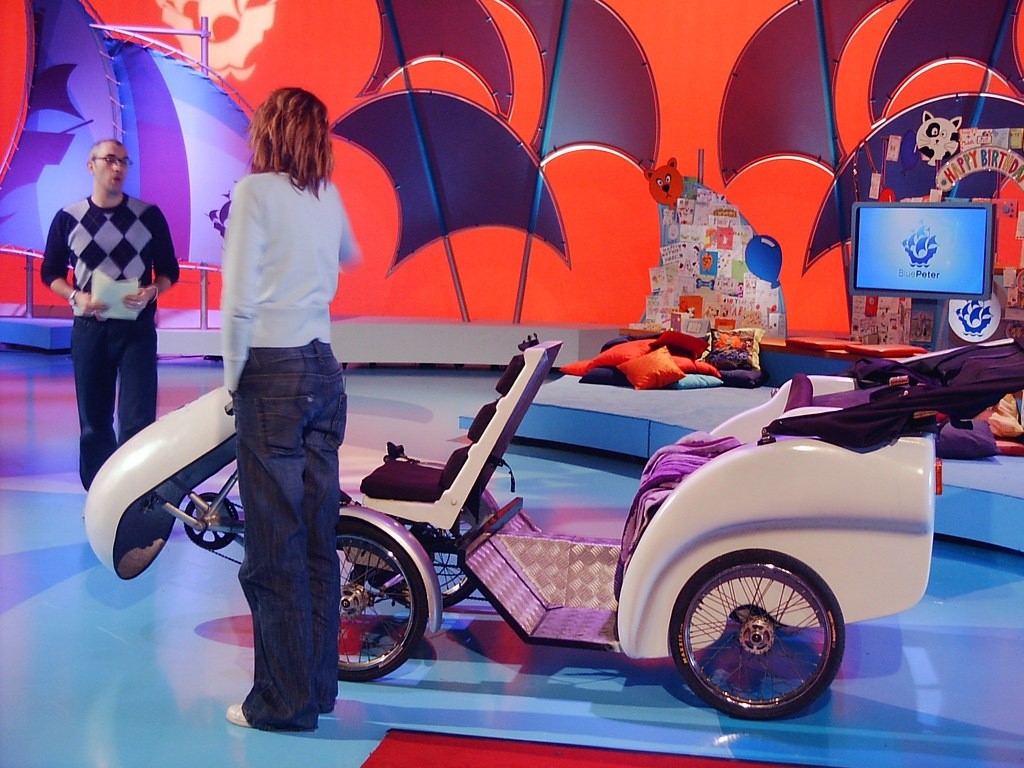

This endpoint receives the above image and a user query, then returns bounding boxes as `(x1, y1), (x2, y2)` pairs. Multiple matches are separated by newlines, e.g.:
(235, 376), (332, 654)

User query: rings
(91, 311), (93, 315)
(139, 300), (143, 305)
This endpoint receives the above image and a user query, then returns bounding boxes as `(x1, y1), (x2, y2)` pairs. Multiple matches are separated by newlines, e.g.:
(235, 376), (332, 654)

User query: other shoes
(225, 703), (251, 728)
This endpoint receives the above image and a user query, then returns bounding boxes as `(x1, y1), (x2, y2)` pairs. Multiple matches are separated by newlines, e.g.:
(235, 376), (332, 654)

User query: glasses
(93, 154), (133, 166)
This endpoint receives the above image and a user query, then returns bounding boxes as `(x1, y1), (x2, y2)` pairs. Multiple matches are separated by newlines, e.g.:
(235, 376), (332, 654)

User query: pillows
(559, 327), (767, 390)
(932, 394), (1024, 459)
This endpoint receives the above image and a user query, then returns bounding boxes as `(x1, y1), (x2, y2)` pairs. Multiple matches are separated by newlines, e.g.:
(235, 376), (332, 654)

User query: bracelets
(150, 283), (159, 303)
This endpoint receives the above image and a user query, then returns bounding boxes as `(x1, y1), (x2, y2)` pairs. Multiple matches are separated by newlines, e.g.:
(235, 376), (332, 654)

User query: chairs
(617, 376), (937, 659)
(359, 339), (563, 530)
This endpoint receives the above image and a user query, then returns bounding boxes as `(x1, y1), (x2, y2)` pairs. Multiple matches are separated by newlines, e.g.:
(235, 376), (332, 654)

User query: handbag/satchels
(359, 458), (445, 503)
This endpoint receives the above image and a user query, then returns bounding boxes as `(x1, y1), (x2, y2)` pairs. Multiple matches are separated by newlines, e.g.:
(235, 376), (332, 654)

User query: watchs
(69, 290), (78, 305)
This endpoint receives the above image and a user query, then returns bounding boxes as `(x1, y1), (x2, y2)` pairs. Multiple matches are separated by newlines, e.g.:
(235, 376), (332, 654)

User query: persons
(39, 139), (180, 518)
(222, 87), (350, 734)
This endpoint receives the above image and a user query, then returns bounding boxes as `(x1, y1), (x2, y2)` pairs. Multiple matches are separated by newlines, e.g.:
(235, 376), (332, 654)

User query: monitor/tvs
(850, 202), (993, 301)
